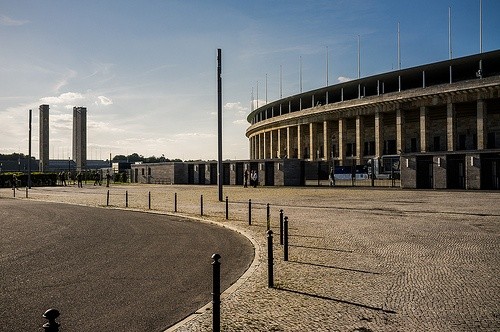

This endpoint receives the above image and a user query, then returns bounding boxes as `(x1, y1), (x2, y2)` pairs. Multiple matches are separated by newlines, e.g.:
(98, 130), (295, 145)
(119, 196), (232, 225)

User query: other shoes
(106, 185), (109, 187)
(94, 183), (96, 186)
(78, 185), (82, 187)
(99, 184), (102, 185)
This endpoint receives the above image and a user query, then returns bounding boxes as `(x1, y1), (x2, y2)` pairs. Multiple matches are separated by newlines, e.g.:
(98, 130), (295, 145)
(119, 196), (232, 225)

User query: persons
(106, 170), (111, 187)
(76, 172), (84, 188)
(250, 169), (258, 188)
(94, 171), (102, 186)
(59, 172), (71, 187)
(243, 170), (249, 188)
